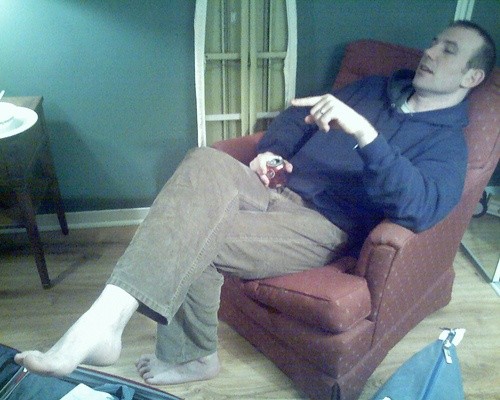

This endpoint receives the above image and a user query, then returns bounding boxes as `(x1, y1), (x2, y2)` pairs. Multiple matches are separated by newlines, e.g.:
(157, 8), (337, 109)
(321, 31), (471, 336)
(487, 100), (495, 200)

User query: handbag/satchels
(370, 326), (466, 400)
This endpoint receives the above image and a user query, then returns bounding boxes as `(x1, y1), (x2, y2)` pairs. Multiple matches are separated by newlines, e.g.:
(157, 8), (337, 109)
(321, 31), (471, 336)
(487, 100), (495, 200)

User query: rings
(320, 109), (324, 116)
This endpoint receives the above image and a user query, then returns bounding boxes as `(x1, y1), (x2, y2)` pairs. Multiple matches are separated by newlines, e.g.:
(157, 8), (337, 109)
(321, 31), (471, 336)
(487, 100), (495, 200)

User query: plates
(0, 107), (38, 139)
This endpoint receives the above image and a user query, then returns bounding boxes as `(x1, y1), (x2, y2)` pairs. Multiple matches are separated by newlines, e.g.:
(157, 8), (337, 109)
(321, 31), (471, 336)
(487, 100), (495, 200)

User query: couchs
(210, 38), (500, 400)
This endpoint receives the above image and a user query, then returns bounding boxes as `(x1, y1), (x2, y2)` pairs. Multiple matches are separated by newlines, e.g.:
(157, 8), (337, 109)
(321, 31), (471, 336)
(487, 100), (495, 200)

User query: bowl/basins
(0, 102), (16, 130)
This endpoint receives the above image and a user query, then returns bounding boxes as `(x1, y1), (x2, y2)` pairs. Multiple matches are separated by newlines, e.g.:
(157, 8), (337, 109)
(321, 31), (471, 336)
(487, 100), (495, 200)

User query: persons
(14, 20), (495, 384)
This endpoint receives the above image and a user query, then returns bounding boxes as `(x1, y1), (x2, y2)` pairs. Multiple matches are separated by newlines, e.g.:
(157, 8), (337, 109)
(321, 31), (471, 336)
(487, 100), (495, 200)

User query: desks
(0, 95), (69, 289)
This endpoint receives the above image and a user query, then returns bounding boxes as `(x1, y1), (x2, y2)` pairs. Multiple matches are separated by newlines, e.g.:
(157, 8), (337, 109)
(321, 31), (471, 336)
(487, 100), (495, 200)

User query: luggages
(0, 343), (196, 400)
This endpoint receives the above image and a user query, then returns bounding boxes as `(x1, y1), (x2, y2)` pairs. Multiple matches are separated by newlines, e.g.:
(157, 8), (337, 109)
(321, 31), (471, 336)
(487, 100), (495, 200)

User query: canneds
(266, 158), (286, 188)
